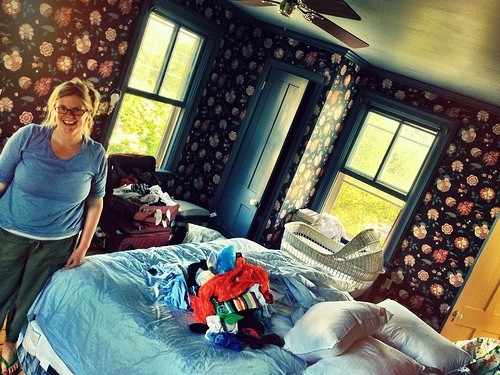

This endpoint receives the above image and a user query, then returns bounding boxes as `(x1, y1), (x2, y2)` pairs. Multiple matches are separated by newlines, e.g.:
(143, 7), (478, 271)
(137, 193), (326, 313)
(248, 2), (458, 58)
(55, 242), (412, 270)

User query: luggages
(105, 154), (180, 225)
(93, 207), (172, 253)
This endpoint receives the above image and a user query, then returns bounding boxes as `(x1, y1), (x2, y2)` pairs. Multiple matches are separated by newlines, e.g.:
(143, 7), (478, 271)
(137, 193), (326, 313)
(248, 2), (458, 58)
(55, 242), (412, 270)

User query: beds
(280, 221), (385, 297)
(16, 238), (500, 375)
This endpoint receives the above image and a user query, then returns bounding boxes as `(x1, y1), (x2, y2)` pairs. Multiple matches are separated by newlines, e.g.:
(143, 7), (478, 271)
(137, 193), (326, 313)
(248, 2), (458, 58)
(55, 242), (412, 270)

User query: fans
(234, 0), (370, 49)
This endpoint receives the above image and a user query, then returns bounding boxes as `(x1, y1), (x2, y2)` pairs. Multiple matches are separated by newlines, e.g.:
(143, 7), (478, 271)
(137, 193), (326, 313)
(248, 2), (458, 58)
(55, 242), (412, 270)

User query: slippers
(0, 354), (21, 375)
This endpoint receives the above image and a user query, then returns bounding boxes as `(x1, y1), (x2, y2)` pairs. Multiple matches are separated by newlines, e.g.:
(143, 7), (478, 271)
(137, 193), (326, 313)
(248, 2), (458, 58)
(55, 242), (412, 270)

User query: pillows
(285, 298), (476, 375)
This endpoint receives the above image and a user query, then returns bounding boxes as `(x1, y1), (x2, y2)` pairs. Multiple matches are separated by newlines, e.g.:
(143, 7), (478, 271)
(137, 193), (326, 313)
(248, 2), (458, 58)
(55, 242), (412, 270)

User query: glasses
(53, 104), (88, 116)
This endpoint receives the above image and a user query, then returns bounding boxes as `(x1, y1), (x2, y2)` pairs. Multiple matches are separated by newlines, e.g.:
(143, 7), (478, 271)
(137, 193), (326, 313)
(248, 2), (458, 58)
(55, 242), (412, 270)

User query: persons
(0, 80), (108, 375)
